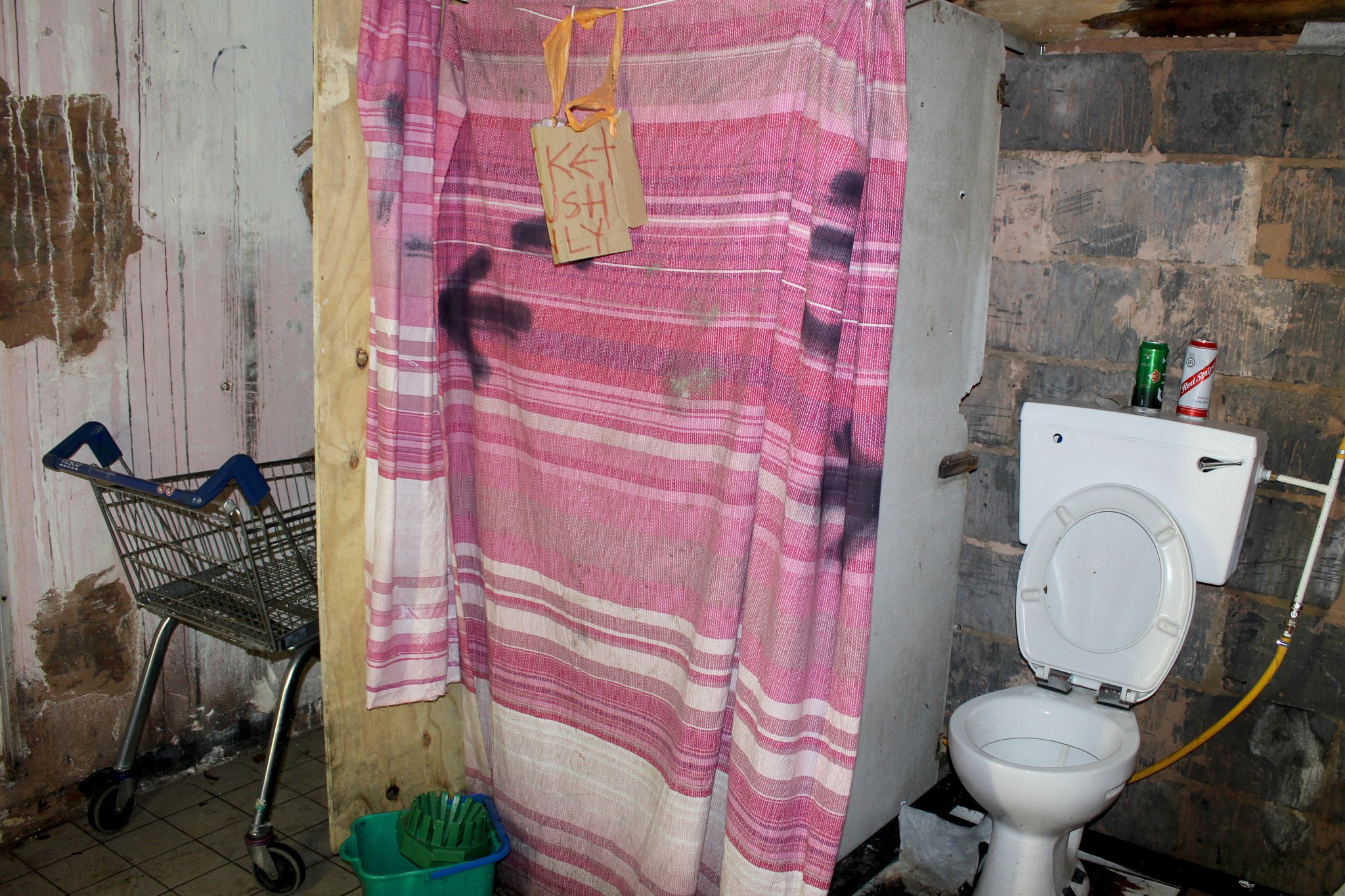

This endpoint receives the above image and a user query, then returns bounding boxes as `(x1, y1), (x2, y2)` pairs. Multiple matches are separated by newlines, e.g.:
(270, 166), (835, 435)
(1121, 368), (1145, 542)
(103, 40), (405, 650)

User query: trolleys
(42, 419), (320, 894)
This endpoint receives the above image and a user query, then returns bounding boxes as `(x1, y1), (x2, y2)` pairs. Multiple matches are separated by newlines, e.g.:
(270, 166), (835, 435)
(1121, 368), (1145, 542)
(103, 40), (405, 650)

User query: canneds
(1176, 338), (1219, 421)
(1130, 339), (1170, 414)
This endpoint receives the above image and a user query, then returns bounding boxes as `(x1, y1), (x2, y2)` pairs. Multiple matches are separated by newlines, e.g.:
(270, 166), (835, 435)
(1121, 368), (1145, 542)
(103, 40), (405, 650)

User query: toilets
(943, 391), (1269, 896)
